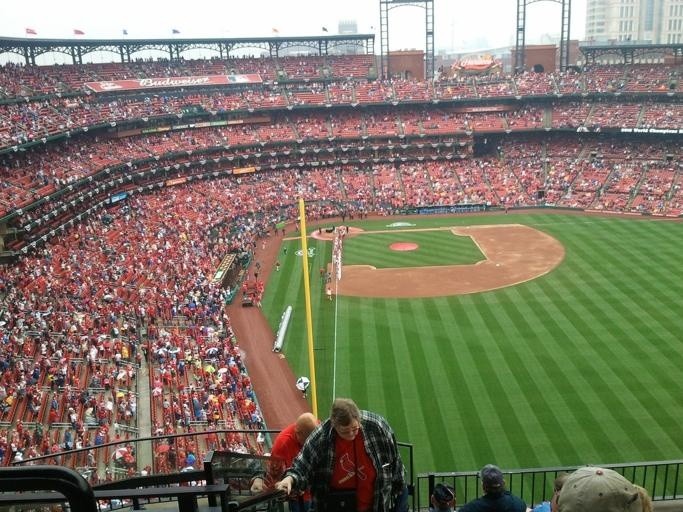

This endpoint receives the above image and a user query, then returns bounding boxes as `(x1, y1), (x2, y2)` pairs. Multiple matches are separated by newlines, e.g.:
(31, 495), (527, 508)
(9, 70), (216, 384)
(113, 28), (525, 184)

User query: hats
(477, 463), (505, 485)
(557, 466), (644, 512)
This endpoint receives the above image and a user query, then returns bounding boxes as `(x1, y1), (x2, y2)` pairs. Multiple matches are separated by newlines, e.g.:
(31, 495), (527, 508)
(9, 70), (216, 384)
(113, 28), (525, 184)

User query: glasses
(336, 422), (361, 434)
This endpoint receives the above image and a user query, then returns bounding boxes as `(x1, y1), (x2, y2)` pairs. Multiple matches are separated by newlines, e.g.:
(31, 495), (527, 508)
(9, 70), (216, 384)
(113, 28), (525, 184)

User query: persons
(0, 59), (683, 218)
(455, 463), (526, 512)
(525, 471), (567, 512)
(273, 397), (411, 511)
(0, 216), (351, 511)
(429, 480), (455, 512)
